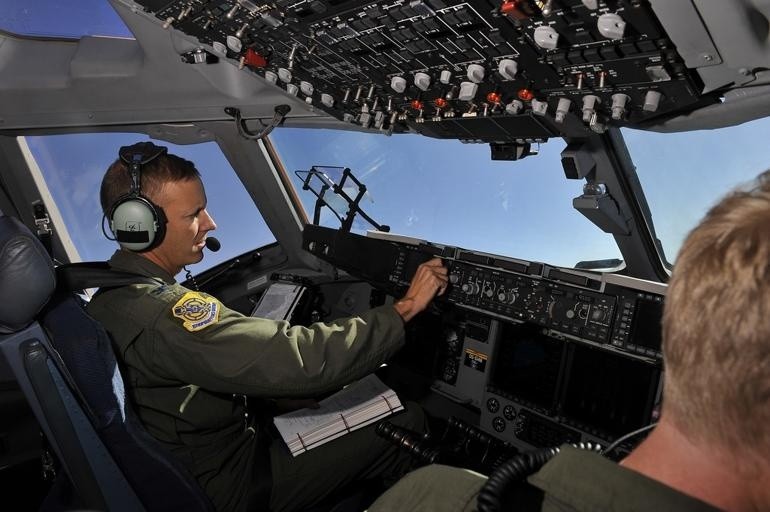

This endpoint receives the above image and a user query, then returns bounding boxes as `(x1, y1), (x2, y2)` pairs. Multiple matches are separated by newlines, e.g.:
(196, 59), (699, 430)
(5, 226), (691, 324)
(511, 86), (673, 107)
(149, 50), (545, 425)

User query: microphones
(206, 235), (223, 254)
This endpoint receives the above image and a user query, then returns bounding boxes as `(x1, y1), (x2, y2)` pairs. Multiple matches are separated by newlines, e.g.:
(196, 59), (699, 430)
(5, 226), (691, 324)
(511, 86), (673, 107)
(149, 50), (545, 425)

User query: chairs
(0, 214), (215, 511)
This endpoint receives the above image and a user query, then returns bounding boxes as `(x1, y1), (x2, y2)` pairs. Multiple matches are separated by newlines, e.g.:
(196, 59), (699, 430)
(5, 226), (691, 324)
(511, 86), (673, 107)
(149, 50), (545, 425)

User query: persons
(362, 166), (770, 512)
(82, 143), (448, 512)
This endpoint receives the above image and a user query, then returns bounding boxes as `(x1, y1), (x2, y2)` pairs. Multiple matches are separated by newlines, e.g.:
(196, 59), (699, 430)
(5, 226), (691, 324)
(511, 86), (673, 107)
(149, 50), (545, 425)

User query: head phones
(101, 143), (167, 255)
